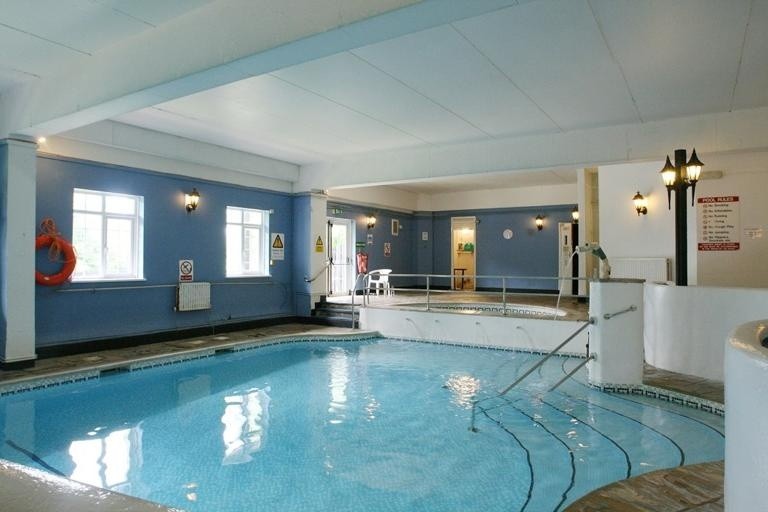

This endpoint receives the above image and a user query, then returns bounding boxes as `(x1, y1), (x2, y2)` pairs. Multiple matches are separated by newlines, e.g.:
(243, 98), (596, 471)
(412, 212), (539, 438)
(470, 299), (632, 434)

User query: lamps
(185, 187), (201, 211)
(367, 213), (376, 228)
(535, 215), (545, 230)
(633, 192), (647, 216)
(570, 206), (578, 223)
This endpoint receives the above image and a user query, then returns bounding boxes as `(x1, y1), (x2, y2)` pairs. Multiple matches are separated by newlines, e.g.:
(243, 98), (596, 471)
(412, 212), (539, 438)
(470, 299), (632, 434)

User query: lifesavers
(34, 233), (78, 287)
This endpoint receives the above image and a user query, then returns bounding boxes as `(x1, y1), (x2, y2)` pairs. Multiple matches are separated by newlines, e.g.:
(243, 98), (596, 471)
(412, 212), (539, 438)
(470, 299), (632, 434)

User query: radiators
(173, 282), (212, 312)
(607, 257), (671, 283)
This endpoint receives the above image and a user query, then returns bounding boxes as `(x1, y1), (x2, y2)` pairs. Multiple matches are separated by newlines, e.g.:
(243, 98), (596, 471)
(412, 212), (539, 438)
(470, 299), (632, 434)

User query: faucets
(575, 240), (610, 280)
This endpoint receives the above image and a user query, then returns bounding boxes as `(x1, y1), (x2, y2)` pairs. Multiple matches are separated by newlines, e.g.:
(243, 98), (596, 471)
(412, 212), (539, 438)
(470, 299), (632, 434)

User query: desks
(454, 268), (469, 290)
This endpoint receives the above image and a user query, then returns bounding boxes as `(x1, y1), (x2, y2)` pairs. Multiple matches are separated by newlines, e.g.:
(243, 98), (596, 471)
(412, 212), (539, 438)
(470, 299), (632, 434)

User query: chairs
(364, 269), (392, 297)
